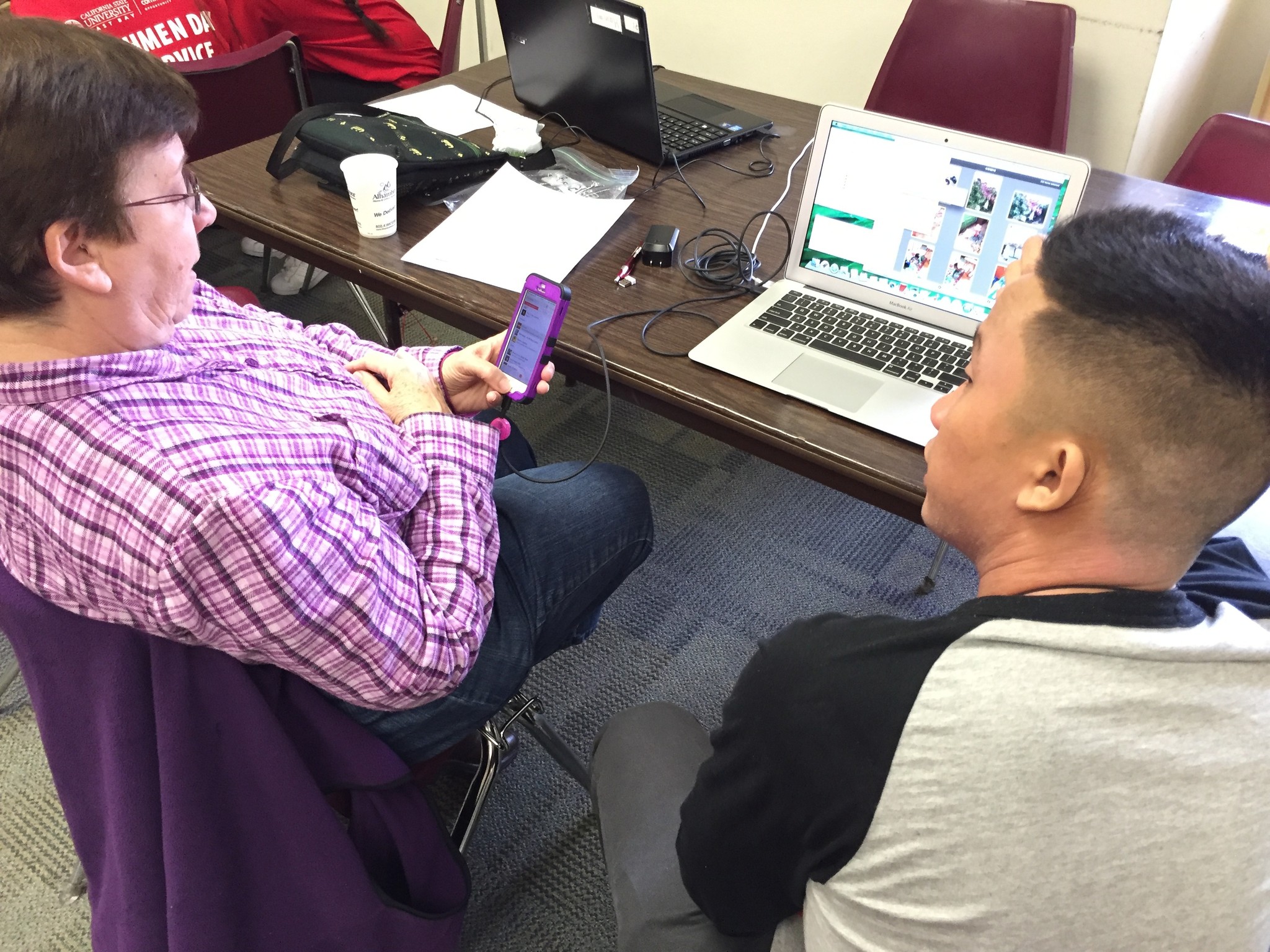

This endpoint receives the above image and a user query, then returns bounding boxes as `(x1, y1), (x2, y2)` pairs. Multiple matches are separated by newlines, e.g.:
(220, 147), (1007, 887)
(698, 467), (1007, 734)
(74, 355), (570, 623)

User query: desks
(185, 54), (1270, 577)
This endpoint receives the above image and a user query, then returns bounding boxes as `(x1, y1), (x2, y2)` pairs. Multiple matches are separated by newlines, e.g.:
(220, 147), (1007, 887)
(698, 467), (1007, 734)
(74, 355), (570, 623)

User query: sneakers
(271, 255), (329, 295)
(241, 234), (285, 258)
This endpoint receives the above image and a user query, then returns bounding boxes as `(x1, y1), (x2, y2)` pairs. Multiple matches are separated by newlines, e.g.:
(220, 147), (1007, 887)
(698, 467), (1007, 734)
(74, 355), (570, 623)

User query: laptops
(496, 0), (773, 166)
(688, 103), (1091, 447)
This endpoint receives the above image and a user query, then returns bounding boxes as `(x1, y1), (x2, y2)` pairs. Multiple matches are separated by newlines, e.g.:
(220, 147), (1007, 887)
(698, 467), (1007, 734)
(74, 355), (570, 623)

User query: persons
(0, 13), (653, 763)
(230, 0), (442, 102)
(585, 201), (1270, 952)
(910, 253), (921, 265)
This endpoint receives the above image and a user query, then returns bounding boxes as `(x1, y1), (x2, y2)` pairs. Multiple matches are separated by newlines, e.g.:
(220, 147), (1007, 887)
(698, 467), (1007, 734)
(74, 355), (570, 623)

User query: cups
(340, 153), (399, 238)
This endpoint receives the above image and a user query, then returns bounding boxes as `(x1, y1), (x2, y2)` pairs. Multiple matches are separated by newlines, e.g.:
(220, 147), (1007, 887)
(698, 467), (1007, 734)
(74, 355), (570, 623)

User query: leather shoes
(420, 725), (518, 782)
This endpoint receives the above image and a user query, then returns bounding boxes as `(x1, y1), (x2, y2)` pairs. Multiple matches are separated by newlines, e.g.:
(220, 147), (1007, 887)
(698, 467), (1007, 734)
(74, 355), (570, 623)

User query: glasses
(39, 168), (200, 254)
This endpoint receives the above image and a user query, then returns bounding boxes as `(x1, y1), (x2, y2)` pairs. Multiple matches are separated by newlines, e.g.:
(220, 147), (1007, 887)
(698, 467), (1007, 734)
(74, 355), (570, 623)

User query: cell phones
(495, 273), (572, 405)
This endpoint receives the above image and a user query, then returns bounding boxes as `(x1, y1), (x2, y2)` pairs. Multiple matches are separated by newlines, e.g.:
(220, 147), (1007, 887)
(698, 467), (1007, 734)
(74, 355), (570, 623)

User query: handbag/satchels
(265, 100), (552, 202)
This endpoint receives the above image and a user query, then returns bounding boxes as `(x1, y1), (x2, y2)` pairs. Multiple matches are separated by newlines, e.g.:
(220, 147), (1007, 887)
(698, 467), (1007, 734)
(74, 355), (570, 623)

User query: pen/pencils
(615, 239), (645, 282)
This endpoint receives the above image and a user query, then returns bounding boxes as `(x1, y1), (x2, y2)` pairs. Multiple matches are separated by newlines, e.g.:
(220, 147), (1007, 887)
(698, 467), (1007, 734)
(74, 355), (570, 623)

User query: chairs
(861, 1), (1078, 591)
(1163, 113), (1270, 206)
(0, 559), (592, 903)
(165, 31), (388, 350)
(440, 0), (464, 77)
